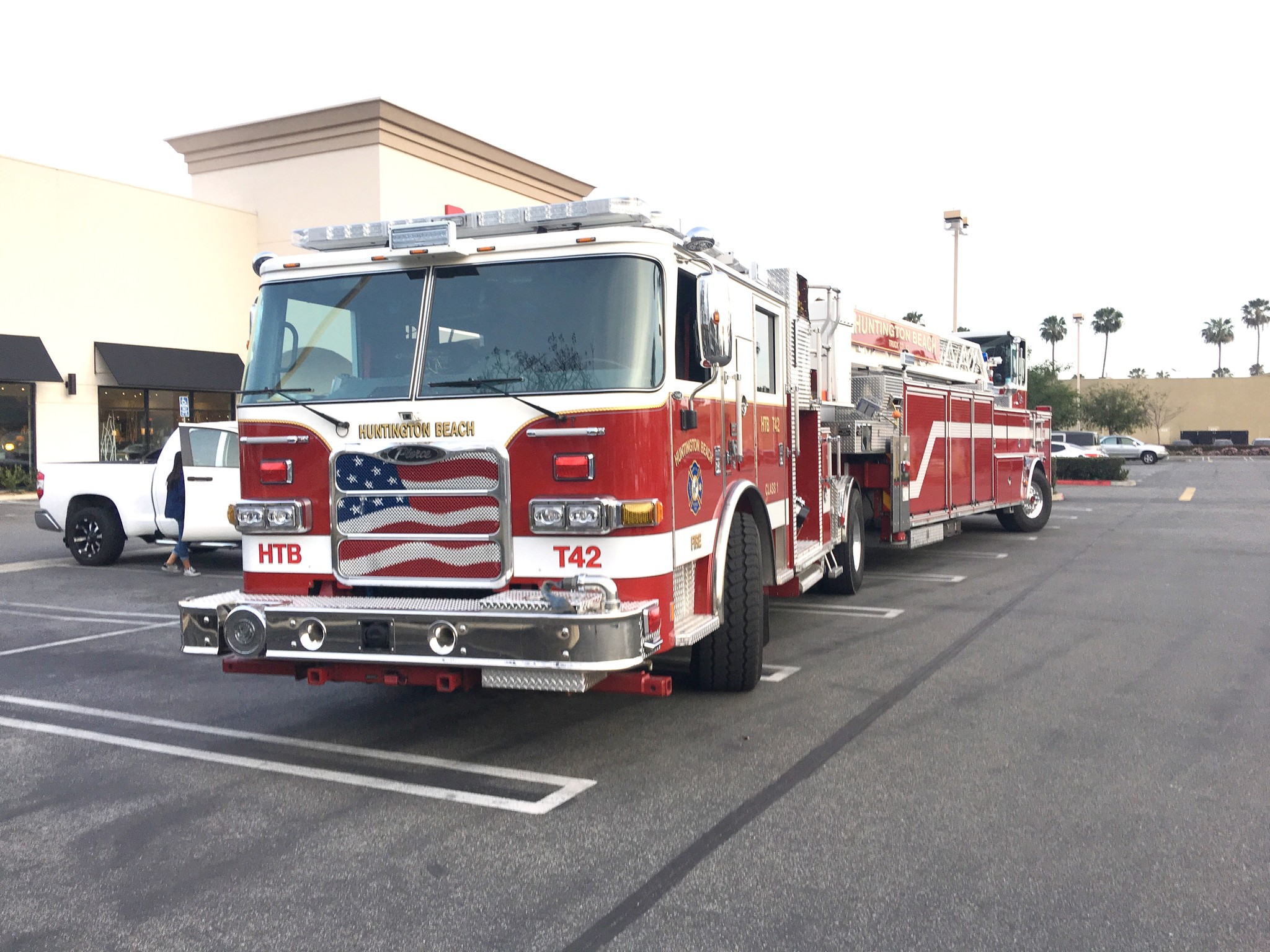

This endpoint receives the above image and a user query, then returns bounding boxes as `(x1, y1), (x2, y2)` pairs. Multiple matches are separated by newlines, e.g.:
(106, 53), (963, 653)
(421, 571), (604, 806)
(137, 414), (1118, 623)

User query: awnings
(94, 342), (245, 393)
(0, 334), (64, 383)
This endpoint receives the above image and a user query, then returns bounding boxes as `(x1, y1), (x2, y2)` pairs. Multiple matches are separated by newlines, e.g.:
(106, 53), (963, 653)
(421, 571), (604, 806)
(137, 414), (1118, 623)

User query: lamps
(65, 374), (76, 395)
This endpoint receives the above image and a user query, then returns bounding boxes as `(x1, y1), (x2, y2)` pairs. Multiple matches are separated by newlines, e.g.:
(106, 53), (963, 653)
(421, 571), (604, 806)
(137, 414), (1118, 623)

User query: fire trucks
(175, 196), (1055, 697)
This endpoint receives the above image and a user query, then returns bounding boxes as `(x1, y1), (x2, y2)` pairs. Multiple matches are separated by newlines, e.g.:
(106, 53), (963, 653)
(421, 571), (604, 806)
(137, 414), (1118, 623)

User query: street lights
(943, 208), (970, 333)
(1073, 313), (1086, 432)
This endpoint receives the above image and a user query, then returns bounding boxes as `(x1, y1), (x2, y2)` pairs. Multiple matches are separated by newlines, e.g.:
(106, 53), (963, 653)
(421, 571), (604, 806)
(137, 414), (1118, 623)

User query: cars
(1050, 442), (1105, 458)
(1099, 435), (1168, 465)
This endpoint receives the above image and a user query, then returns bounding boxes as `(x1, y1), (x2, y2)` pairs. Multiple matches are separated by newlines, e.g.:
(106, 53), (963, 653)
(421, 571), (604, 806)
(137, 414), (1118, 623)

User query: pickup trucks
(34, 420), (242, 568)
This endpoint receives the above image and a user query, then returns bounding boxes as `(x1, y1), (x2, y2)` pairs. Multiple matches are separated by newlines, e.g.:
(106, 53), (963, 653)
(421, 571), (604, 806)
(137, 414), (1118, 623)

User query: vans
(1051, 430), (1098, 446)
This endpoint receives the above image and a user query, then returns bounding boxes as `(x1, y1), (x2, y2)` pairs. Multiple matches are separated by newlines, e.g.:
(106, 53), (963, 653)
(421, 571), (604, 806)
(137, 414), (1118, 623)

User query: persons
(161, 451), (201, 577)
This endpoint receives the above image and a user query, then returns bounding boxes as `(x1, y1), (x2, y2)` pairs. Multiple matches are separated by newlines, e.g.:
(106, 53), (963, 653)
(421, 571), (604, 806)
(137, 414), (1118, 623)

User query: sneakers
(183, 565), (201, 577)
(162, 563), (181, 572)
